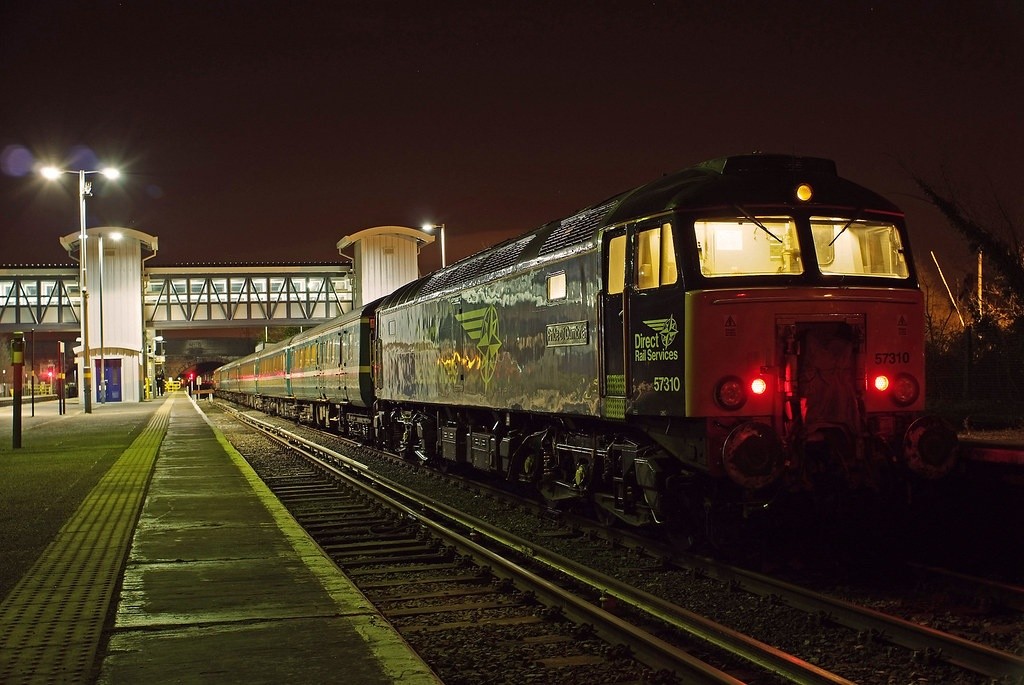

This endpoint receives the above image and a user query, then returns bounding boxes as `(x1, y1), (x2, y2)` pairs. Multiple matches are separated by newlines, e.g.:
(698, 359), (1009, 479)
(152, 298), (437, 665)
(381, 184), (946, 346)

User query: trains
(212, 152), (959, 563)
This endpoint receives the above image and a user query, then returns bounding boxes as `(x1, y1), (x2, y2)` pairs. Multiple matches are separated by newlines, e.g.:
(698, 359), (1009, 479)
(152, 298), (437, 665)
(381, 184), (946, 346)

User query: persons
(161, 369), (165, 393)
(155, 371), (164, 396)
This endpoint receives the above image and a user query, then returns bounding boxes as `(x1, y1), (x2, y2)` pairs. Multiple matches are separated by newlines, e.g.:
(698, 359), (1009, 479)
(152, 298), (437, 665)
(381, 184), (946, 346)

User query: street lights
(421, 223), (446, 268)
(78, 231), (124, 404)
(40, 166), (120, 414)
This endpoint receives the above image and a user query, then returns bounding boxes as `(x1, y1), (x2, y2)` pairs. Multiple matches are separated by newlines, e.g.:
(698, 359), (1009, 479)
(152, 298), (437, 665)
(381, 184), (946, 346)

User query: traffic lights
(47, 366), (53, 376)
